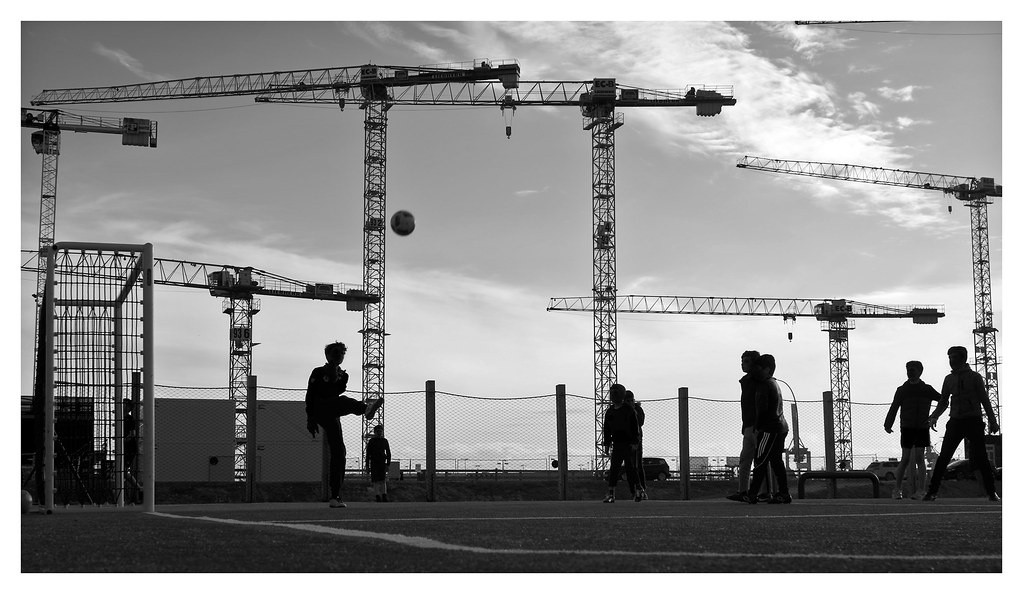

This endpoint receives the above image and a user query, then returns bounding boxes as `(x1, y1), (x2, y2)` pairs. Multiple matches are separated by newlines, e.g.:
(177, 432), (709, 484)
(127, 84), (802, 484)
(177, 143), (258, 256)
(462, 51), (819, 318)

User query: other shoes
(990, 492), (1000, 501)
(726, 491), (747, 502)
(923, 490), (938, 500)
(767, 492), (792, 504)
(603, 494), (614, 503)
(377, 494), (387, 502)
(364, 397), (384, 419)
(329, 495), (346, 507)
(757, 492), (773, 502)
(892, 489), (903, 499)
(635, 489), (644, 501)
(643, 492), (648, 500)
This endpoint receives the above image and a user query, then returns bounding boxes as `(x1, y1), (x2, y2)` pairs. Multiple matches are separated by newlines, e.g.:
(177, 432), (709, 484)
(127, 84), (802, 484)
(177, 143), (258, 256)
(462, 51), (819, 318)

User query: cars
(944, 459), (1002, 482)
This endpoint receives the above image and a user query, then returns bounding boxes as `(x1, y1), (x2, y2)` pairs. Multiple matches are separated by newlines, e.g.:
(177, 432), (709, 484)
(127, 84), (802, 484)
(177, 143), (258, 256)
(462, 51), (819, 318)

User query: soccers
(391, 211), (415, 235)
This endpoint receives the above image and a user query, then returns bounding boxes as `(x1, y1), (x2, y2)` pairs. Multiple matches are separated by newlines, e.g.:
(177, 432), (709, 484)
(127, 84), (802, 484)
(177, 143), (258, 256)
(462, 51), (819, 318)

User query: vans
(866, 461), (908, 480)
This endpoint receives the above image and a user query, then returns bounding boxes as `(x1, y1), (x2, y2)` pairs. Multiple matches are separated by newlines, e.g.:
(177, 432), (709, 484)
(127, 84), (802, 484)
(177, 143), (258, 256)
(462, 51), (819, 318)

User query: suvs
(621, 458), (671, 481)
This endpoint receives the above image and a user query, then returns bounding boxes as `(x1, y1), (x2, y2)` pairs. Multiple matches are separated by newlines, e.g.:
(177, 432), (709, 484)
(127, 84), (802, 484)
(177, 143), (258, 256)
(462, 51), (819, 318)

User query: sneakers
(736, 493), (759, 504)
(911, 491), (926, 500)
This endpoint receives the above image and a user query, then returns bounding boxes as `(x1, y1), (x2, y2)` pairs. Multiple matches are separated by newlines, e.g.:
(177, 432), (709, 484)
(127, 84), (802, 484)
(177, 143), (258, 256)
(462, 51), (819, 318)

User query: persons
(884, 346), (1000, 502)
(726, 350), (793, 504)
(603, 384), (649, 503)
(365, 425), (391, 503)
(305, 342), (385, 508)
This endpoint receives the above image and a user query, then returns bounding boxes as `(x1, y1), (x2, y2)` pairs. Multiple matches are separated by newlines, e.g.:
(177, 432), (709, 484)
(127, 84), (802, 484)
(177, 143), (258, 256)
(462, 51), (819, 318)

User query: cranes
(21, 106), (163, 400)
(21, 249), (381, 482)
(551, 297), (944, 475)
(254, 73), (733, 472)
(745, 154), (1002, 442)
(59, 56), (518, 475)
